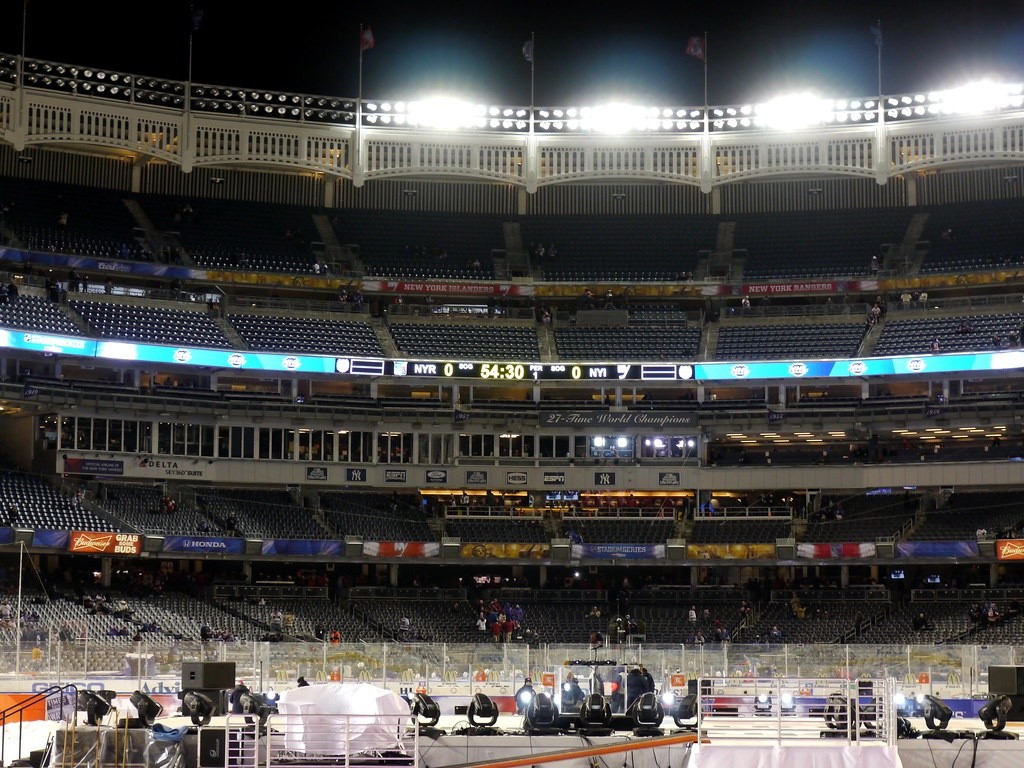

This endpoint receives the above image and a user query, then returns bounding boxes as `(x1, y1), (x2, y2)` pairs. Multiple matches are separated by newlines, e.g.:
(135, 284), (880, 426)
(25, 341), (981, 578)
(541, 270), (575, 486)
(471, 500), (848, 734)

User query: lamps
(184, 691), (216, 726)
(579, 692), (614, 736)
(633, 692), (665, 737)
(240, 693), (272, 732)
(406, 693), (447, 735)
(523, 693), (560, 735)
(467, 693), (504, 736)
(820, 693), (865, 741)
(861, 696), (905, 738)
(78, 690), (111, 726)
(921, 695), (959, 739)
(670, 693), (707, 735)
(130, 690), (163, 728)
(754, 694), (772, 717)
(977, 694), (1019, 740)
(781, 693), (797, 716)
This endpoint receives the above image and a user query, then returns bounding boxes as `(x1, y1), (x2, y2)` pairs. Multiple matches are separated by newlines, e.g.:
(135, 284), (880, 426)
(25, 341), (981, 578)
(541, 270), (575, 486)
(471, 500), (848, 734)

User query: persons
(514, 677), (536, 715)
(0, 483), (1023, 675)
(561, 677), (584, 713)
(589, 664), (655, 713)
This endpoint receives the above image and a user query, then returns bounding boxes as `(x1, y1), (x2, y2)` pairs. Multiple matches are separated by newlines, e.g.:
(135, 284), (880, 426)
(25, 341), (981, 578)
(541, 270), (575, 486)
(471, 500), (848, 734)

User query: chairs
(0, 202), (1023, 768)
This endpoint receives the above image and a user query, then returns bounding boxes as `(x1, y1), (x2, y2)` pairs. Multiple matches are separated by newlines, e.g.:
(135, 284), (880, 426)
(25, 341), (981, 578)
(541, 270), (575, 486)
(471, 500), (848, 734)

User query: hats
(633, 665), (640, 671)
(297, 676), (304, 683)
(564, 670), (574, 678)
(525, 677), (531, 686)
(207, 623), (210, 626)
(239, 680), (243, 685)
